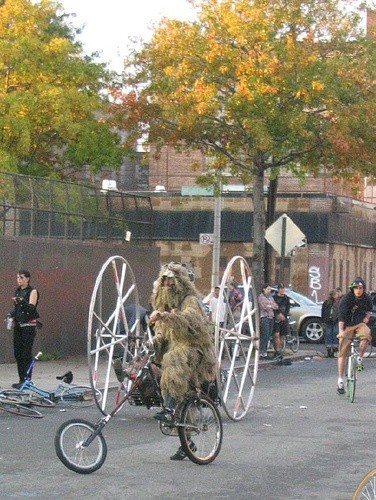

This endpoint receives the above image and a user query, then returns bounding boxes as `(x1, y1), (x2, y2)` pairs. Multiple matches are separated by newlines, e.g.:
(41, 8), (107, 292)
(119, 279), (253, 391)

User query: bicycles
(337, 335), (372, 404)
(351, 468), (376, 500)
(274, 315), (299, 353)
(54, 312), (224, 474)
(0, 352), (102, 418)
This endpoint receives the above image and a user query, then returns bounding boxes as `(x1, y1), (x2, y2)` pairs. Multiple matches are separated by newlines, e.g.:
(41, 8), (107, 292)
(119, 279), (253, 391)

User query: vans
(202, 286), (326, 343)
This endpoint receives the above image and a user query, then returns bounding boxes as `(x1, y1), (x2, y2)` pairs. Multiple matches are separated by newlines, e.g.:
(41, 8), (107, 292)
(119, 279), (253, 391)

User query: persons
(150, 262), (217, 461)
(258, 284), (278, 361)
(272, 283), (288, 359)
(208, 281), (242, 360)
(5, 270), (40, 388)
(337, 277), (372, 394)
(321, 290), (339, 358)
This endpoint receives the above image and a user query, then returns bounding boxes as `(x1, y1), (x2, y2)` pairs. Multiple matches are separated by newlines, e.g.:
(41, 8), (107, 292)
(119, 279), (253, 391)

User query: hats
(162, 268), (174, 278)
(329, 289), (337, 297)
(348, 281), (364, 287)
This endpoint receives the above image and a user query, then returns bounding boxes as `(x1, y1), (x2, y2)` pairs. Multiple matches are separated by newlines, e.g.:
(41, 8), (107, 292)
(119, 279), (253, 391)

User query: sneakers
(357, 357), (363, 372)
(170, 441), (197, 460)
(259, 355), (274, 361)
(337, 381), (345, 397)
(153, 409), (175, 424)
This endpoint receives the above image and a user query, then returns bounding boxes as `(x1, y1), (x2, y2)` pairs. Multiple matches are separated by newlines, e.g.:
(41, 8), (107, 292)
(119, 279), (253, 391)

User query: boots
(326, 346), (339, 358)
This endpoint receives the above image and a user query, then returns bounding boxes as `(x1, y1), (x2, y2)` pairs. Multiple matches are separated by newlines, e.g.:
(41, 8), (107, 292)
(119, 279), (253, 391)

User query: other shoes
(274, 351), (284, 359)
(11, 383), (34, 388)
